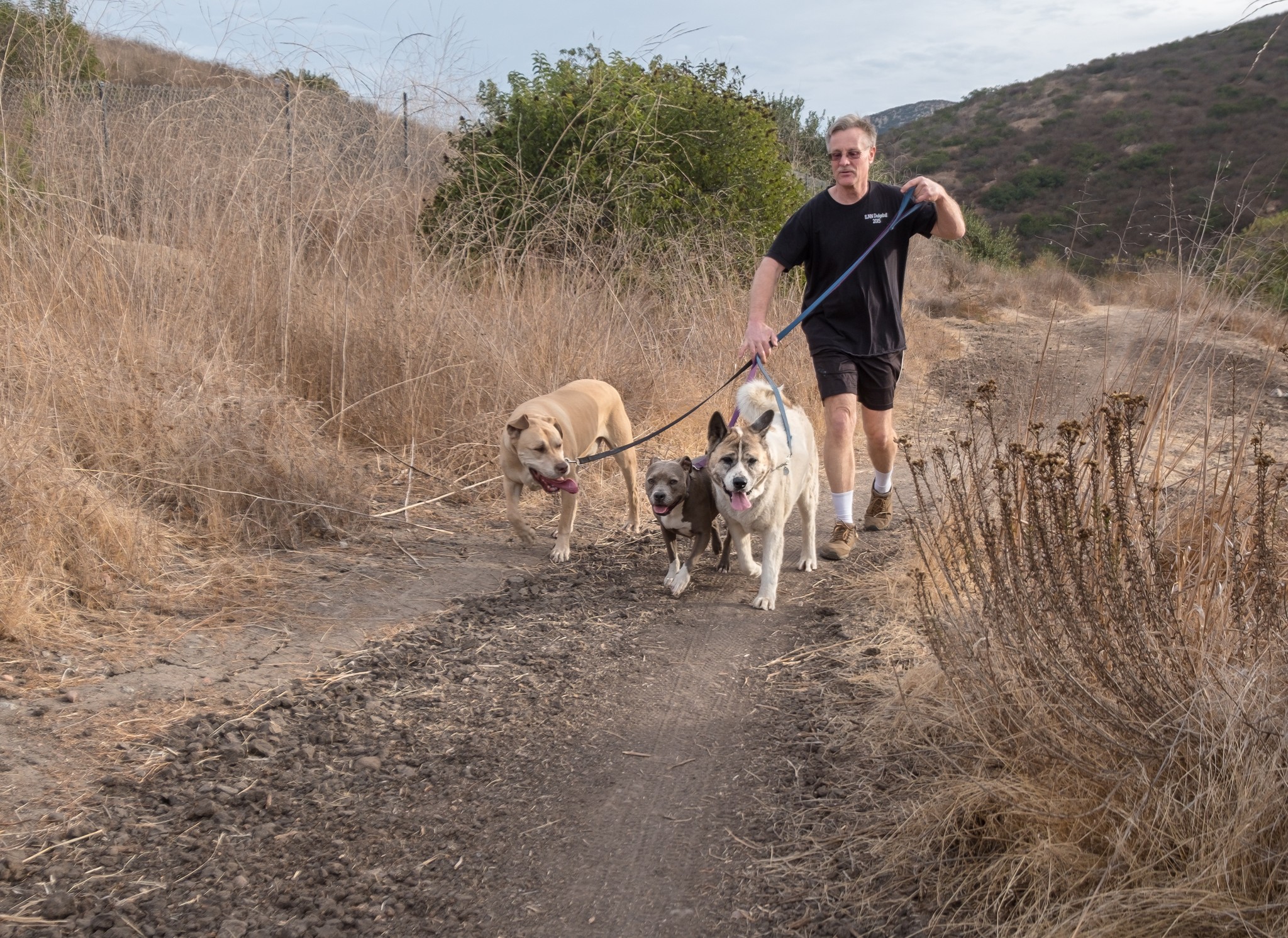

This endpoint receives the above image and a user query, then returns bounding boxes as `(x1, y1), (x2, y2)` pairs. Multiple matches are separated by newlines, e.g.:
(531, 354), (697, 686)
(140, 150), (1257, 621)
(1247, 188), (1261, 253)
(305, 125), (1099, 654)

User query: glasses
(828, 146), (872, 161)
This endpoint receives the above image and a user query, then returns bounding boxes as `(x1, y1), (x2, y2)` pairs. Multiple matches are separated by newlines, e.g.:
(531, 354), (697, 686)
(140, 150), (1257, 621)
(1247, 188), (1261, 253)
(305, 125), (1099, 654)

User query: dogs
(499, 378), (641, 564)
(704, 382), (820, 612)
(646, 455), (733, 598)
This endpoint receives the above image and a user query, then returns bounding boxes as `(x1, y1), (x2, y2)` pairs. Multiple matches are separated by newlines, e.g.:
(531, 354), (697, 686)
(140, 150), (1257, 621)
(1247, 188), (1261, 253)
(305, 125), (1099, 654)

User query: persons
(739, 114), (965, 559)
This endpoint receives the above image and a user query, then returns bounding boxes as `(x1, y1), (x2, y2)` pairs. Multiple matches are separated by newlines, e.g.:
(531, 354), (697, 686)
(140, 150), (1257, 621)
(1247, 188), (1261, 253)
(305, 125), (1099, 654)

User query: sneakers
(864, 475), (894, 530)
(820, 519), (857, 560)
